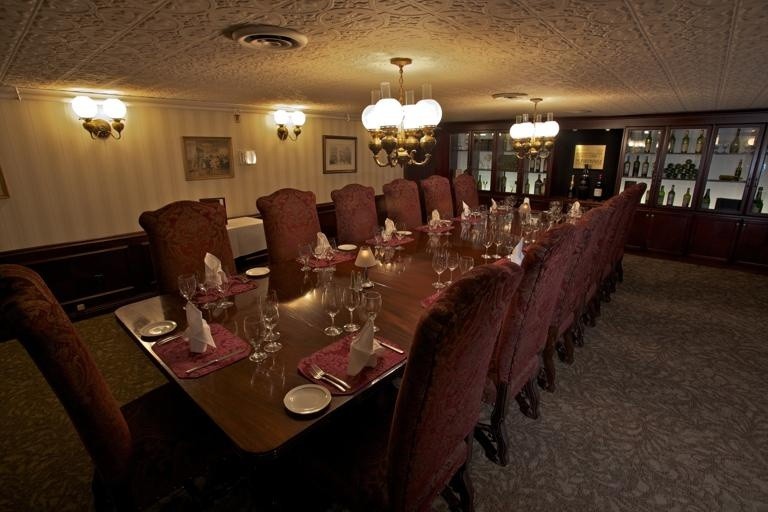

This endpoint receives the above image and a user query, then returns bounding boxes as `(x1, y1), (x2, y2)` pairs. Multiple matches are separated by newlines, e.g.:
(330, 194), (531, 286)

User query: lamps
(72, 94), (127, 140)
(275, 110), (305, 144)
(361, 58), (442, 167)
(510, 98), (560, 160)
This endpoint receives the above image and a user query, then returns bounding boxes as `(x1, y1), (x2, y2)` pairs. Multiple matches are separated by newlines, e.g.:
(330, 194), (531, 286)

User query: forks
(296, 361), (352, 392)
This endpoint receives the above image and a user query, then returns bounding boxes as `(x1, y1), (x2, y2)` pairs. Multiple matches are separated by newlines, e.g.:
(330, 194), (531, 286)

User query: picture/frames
(199, 197), (228, 225)
(322, 135), (358, 174)
(181, 136), (235, 181)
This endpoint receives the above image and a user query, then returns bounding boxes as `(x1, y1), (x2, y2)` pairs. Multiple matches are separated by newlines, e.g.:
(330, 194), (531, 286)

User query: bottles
(701, 188), (710, 209)
(524, 178), (530, 196)
(623, 154), (630, 177)
(567, 174), (575, 198)
(597, 172), (605, 182)
(662, 160), (697, 179)
(658, 185), (665, 205)
(695, 129), (705, 153)
(534, 174), (542, 196)
(641, 155), (649, 178)
(681, 130), (690, 153)
(734, 158), (743, 180)
(478, 175), (482, 191)
(632, 155), (640, 177)
(719, 175), (744, 181)
(730, 128), (741, 153)
(668, 130), (676, 153)
(541, 178), (547, 195)
(578, 174), (588, 199)
(645, 131), (651, 153)
(752, 187), (763, 214)
(582, 163), (590, 175)
(593, 182), (604, 201)
(666, 184), (675, 206)
(682, 188), (691, 207)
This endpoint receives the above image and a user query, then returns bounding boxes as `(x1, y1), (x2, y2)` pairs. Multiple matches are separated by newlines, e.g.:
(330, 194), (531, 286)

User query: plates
(245, 267), (270, 277)
(282, 383), (332, 416)
(139, 319), (178, 338)
(337, 244), (357, 251)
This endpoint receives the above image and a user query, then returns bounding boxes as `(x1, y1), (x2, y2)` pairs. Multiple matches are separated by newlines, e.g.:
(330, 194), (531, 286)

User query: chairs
(542, 205), (618, 393)
(476, 222), (580, 467)
(594, 186), (637, 318)
(138, 200), (238, 293)
(1, 264), (297, 512)
(421, 175), (454, 224)
(610, 182), (647, 293)
(331, 184), (379, 244)
(296, 258), (523, 512)
(575, 194), (629, 347)
(383, 179), (422, 228)
(255, 188), (321, 264)
(452, 173), (479, 216)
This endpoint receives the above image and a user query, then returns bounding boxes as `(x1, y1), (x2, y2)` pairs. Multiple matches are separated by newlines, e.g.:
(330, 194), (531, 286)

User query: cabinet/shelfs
(617, 122), (768, 274)
(449, 128), (555, 210)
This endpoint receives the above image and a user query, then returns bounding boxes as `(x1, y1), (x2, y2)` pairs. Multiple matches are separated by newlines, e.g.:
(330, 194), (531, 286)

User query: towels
(511, 237), (524, 265)
(490, 199), (498, 214)
(522, 197), (530, 212)
(184, 302), (216, 353)
(312, 232), (332, 260)
(571, 200), (582, 219)
(347, 315), (384, 375)
(460, 200), (474, 220)
(382, 218), (396, 242)
(429, 210), (444, 229)
(204, 252), (226, 287)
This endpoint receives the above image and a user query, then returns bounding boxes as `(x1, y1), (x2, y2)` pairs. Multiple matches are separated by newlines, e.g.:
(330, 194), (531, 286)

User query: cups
(259, 290), (281, 341)
(372, 225), (385, 258)
(260, 305), (282, 354)
(341, 287), (361, 333)
(177, 273), (198, 307)
(243, 315), (267, 364)
(442, 217), (454, 228)
(427, 214), (433, 226)
(431, 247), (449, 288)
(211, 265), (236, 309)
(423, 231), (438, 255)
(320, 286), (344, 337)
(392, 222), (407, 252)
(294, 242), (315, 283)
(445, 251), (460, 286)
(196, 268), (213, 308)
(504, 234), (516, 260)
(360, 291), (383, 333)
(492, 231), (504, 260)
(443, 232), (453, 253)
(460, 256), (475, 275)
(481, 228), (493, 260)
(519, 201), (588, 244)
(461, 194), (514, 244)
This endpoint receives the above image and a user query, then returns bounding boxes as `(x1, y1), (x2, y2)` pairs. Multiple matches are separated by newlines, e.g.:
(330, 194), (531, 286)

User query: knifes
(185, 347), (247, 375)
